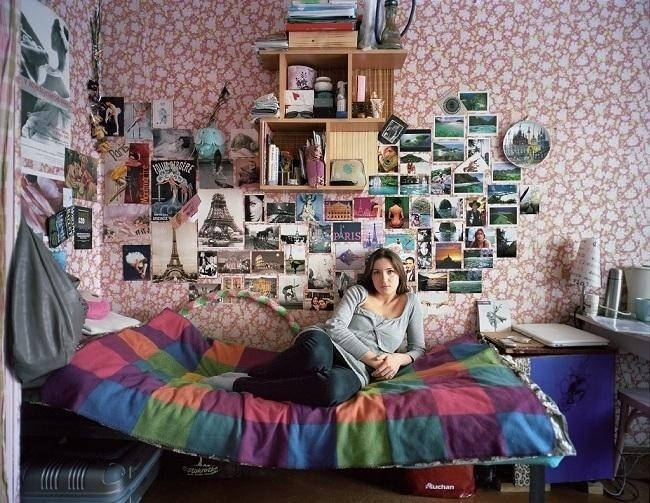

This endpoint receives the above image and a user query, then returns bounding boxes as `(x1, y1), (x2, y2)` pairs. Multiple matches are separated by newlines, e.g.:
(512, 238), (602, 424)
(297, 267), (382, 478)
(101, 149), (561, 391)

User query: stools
(613, 387), (650, 481)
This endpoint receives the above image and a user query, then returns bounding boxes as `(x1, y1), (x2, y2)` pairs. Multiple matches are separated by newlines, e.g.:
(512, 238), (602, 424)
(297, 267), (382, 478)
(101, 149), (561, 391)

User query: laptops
(511, 323), (610, 348)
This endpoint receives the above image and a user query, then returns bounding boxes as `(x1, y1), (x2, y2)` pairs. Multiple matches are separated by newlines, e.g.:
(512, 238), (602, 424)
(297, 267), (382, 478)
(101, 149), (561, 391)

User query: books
(251, 0), (360, 52)
(248, 93), (280, 122)
(266, 144), (280, 186)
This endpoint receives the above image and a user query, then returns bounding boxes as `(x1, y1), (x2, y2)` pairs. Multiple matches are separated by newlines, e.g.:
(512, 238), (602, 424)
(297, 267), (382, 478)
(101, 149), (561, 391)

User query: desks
(570, 309), (649, 363)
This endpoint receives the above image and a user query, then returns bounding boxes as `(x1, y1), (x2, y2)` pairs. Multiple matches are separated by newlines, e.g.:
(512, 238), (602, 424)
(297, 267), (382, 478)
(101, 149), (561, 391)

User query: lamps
(568, 237), (601, 315)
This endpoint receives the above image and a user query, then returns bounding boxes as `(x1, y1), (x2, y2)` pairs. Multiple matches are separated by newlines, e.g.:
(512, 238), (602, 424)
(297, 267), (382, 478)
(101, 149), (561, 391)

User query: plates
(503, 120), (551, 168)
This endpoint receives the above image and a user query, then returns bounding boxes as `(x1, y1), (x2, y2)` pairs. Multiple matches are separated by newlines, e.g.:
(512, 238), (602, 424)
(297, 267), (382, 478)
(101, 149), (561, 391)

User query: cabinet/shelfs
(255, 47), (410, 192)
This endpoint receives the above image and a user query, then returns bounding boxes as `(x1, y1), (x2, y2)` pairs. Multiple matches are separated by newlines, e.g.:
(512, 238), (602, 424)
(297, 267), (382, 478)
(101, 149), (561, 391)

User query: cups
(635, 297), (650, 322)
(584, 293), (599, 316)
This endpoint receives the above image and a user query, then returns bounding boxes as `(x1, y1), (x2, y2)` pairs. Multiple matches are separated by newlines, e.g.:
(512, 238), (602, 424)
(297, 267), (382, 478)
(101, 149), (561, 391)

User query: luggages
(20, 449), (162, 503)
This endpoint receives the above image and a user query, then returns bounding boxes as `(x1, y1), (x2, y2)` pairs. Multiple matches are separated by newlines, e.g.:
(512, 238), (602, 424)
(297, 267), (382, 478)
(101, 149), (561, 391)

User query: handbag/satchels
(7, 215), (88, 385)
(409, 465), (475, 499)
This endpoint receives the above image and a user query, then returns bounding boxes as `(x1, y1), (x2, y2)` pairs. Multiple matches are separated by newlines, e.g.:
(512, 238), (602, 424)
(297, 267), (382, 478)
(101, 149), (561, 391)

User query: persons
(200, 246), (428, 408)
(107, 160), (128, 202)
(388, 196), (406, 229)
(104, 101), (122, 135)
(317, 299), (328, 310)
(250, 195), (264, 222)
(310, 295), (320, 310)
(377, 146), (398, 172)
(282, 284), (301, 304)
(19, 17), (70, 99)
(469, 227), (491, 248)
(406, 158), (416, 174)
(403, 257), (415, 281)
(418, 241), (431, 255)
(125, 151), (144, 203)
(125, 251), (150, 279)
(410, 213), (422, 227)
(466, 200), (482, 226)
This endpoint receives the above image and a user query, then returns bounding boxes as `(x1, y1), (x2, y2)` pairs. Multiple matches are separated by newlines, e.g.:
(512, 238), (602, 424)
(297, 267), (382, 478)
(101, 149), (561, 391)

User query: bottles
(603, 267), (623, 317)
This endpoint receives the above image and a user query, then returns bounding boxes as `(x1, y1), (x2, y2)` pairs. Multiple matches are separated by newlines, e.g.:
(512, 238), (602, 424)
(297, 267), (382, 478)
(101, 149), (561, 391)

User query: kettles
(624, 266), (650, 317)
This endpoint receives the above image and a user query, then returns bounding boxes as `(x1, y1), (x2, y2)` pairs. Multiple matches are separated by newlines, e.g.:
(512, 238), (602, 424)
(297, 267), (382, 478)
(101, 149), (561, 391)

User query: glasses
(287, 111), (313, 118)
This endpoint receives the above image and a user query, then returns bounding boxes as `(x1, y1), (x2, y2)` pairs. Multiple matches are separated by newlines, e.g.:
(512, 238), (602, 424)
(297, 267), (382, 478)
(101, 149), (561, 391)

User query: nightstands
(478, 321), (617, 495)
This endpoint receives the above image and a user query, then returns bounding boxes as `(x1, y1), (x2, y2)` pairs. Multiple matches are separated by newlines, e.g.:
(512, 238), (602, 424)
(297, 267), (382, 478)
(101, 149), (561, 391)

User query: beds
(22, 316), (560, 502)
